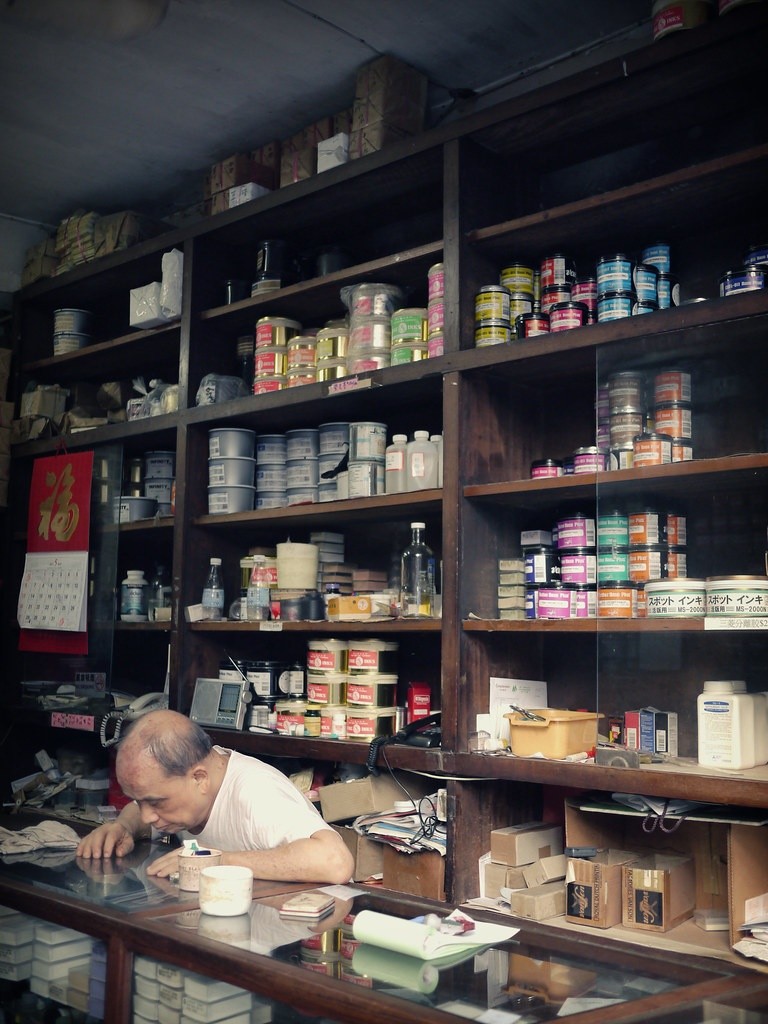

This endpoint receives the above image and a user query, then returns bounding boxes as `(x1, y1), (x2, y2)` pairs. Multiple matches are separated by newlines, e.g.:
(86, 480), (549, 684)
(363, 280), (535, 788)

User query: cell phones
(279, 908), (338, 926)
(279, 894), (336, 917)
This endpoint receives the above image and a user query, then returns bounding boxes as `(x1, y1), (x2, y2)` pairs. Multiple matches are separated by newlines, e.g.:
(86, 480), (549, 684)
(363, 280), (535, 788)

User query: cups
(383, 588), (399, 616)
(198, 866), (253, 916)
(257, 709), (271, 728)
(178, 849), (222, 892)
(391, 707), (406, 735)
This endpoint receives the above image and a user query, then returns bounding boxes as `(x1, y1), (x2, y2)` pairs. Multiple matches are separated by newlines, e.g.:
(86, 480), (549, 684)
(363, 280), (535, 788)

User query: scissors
(510, 705), (546, 721)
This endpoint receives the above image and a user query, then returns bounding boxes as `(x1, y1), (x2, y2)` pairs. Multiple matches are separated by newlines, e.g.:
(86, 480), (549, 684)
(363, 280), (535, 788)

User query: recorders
(189, 657), (254, 730)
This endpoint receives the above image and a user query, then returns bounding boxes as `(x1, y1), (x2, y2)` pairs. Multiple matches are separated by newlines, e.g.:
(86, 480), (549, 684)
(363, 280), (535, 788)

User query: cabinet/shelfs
(0, 804), (768, 1024)
(0, 1), (768, 970)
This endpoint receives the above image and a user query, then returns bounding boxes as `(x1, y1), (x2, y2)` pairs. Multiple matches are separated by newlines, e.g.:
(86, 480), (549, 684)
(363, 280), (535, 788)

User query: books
(351, 943), (491, 993)
(352, 910), (483, 961)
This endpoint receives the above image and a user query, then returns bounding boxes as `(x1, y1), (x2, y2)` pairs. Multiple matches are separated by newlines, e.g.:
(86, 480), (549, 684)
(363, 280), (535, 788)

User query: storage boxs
(0, 906), (272, 1024)
(503, 707), (605, 759)
(128, 281), (172, 331)
(20, 53), (432, 290)
(483, 789), (768, 953)
(318, 772), (446, 902)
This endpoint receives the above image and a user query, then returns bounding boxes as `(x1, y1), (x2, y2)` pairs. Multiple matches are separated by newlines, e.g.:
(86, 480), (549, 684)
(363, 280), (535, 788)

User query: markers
(566, 751), (595, 762)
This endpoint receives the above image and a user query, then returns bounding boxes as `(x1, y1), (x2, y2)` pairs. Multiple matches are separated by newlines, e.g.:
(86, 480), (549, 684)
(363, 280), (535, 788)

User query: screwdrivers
(489, 746), (512, 752)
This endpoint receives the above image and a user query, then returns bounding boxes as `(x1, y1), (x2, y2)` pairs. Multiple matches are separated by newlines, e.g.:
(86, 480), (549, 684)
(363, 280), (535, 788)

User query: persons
(77, 708), (356, 1023)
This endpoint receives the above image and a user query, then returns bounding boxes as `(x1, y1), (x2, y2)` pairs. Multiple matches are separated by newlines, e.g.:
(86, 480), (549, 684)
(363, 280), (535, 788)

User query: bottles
(332, 714), (346, 739)
(323, 584), (342, 621)
(201, 557), (225, 622)
(406, 430), (438, 493)
(147, 567), (173, 622)
(120, 570), (149, 622)
(247, 555), (270, 622)
(304, 705), (321, 737)
(225, 280), (234, 306)
(385, 434), (408, 493)
(256, 242), (281, 281)
(400, 522), (436, 617)
(697, 680), (768, 770)
(430, 431), (444, 488)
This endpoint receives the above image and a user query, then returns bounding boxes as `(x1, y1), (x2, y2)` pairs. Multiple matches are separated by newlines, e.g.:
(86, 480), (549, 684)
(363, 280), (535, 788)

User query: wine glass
(242, 353), (256, 395)
(269, 601), (280, 620)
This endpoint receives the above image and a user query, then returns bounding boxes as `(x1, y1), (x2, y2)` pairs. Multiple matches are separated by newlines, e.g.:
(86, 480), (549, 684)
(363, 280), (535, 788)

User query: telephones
(397, 713), (441, 748)
(126, 692), (164, 718)
